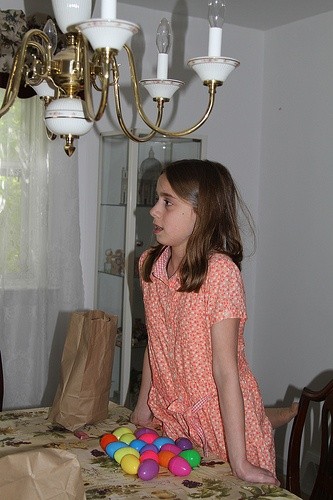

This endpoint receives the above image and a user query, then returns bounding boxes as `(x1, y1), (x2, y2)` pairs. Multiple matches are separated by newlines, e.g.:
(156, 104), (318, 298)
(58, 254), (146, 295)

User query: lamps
(0, 0), (240, 158)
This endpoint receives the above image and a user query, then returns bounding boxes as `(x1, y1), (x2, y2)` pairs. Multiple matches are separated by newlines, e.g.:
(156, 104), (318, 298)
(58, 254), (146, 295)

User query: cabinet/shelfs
(94, 128), (203, 411)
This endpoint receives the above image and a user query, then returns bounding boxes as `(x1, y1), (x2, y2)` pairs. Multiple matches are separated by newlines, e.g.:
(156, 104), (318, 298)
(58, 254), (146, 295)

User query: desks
(0, 400), (304, 500)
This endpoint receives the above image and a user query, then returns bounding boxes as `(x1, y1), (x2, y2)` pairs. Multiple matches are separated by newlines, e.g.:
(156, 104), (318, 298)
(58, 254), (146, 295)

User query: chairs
(286, 380), (333, 500)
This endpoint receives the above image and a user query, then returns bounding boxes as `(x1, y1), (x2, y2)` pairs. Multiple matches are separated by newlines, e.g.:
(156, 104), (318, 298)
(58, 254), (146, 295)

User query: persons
(129, 159), (280, 486)
